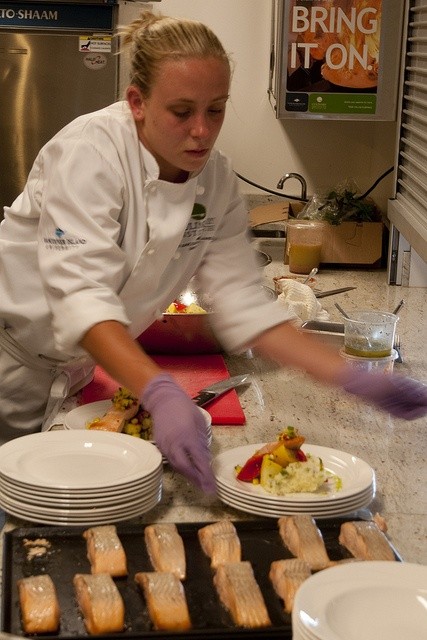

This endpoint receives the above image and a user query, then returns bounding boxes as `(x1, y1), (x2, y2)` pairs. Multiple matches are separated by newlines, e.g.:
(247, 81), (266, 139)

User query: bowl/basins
(252, 245), (272, 280)
(336, 310), (399, 358)
(138, 284), (278, 356)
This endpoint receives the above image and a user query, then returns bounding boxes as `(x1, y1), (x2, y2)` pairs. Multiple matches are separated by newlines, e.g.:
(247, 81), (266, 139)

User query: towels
(274, 279), (330, 326)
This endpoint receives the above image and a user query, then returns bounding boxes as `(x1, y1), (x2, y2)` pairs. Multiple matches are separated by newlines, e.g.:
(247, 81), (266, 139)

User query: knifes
(190, 372), (250, 409)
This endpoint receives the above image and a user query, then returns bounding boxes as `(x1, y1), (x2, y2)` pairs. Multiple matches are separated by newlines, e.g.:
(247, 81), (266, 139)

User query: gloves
(336, 368), (427, 420)
(141, 378), (218, 492)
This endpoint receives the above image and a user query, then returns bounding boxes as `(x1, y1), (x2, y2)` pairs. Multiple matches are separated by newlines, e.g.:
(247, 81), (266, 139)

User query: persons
(1, 10), (427, 496)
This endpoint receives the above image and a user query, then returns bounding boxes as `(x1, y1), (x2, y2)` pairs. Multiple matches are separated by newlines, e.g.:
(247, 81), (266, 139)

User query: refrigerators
(1, 1), (154, 218)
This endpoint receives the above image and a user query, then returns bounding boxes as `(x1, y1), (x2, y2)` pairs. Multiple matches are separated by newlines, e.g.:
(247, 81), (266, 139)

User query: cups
(285, 220), (325, 273)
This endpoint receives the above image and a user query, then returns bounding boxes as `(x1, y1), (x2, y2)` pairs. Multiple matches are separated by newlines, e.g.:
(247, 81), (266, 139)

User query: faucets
(277, 173), (306, 198)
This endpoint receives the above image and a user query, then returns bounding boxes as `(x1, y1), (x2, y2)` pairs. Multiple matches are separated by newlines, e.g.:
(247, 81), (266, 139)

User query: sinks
(246, 229), (286, 238)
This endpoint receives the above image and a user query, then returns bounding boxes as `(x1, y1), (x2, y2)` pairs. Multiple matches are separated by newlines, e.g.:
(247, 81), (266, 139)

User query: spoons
(334, 303), (374, 349)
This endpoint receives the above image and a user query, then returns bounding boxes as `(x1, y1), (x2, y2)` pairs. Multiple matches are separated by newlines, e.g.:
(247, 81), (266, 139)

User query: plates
(3, 428), (162, 526)
(290, 559), (427, 638)
(63, 398), (212, 461)
(216, 447), (373, 521)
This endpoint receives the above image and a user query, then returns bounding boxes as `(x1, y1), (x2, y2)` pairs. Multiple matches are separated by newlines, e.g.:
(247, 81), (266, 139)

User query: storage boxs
(247, 193), (384, 268)
